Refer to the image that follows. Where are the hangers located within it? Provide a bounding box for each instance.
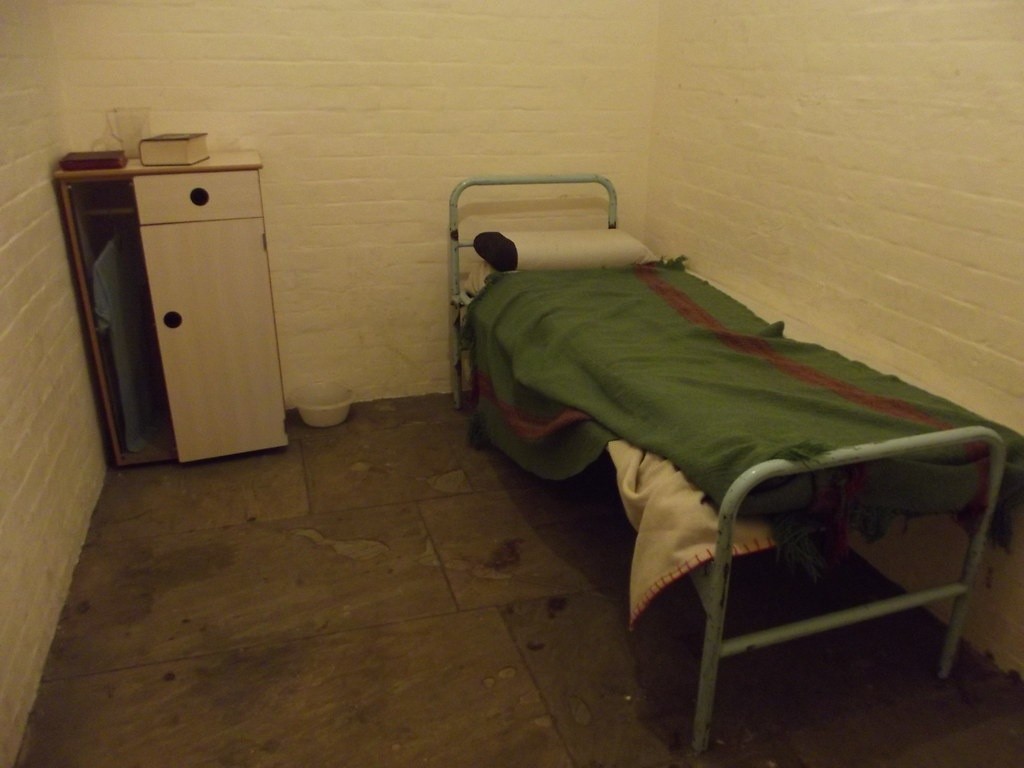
[108,206,124,243]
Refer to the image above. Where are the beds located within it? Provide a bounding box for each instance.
[448,175,1024,755]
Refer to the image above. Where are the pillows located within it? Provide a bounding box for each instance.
[474,229,660,272]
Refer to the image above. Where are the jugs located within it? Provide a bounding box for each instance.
[105,106,154,158]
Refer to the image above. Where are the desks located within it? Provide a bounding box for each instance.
[54,150,289,467]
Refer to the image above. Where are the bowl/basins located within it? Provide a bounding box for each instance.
[289,382,355,427]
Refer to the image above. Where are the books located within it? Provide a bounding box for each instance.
[139,133,210,167]
[59,150,127,170]
